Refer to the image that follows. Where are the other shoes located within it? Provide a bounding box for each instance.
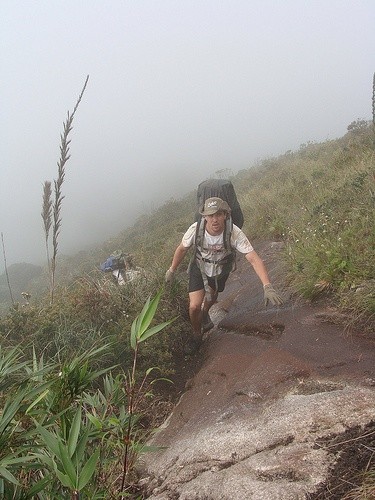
[201,309,214,331]
[184,332,202,354]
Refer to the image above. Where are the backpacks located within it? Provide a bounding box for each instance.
[193,179,244,271]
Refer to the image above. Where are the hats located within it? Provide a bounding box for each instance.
[198,196,232,219]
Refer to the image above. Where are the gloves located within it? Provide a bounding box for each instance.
[262,284,283,306]
[165,269,175,285]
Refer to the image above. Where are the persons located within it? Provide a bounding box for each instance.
[164,196,286,343]
